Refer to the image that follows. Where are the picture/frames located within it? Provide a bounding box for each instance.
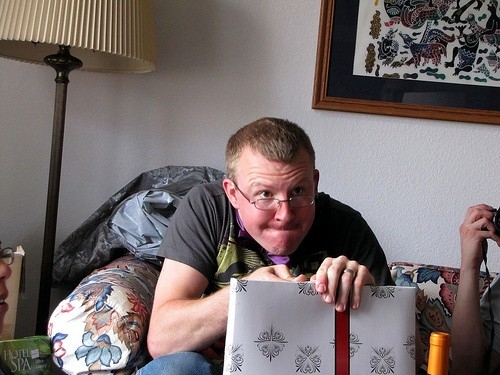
[311,0,500,125]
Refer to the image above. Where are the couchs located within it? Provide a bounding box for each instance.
[46,252,500,375]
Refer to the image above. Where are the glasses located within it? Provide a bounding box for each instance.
[0,247,14,265]
[234,182,316,211]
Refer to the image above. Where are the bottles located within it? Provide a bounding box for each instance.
[427,332,451,375]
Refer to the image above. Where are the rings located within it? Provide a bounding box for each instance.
[344,269,355,278]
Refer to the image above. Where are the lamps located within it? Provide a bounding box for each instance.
[0,0,159,335]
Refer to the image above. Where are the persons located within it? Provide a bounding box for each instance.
[136,118,397,375]
[450,203,500,375]
[0,241,13,375]
